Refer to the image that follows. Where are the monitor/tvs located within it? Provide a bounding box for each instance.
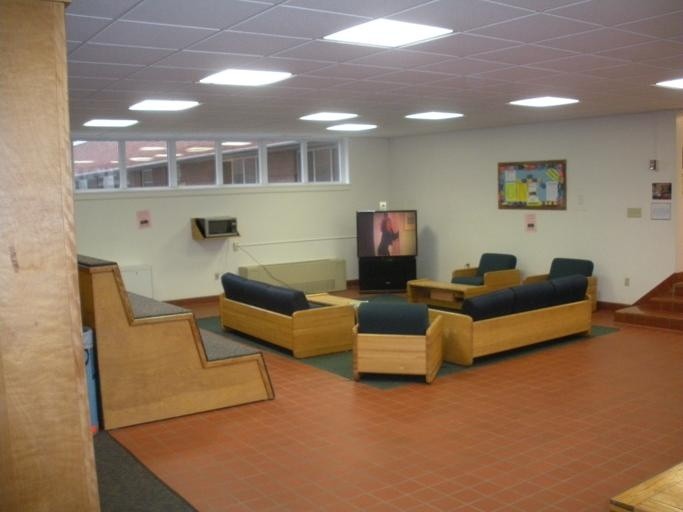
[355,210,418,259]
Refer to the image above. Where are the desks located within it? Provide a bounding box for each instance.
[306,292,358,309]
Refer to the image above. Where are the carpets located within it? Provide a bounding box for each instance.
[94,429,197,512]
[196,294,621,390]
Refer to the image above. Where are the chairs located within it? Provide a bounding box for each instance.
[451,253,521,284]
[522,258,597,312]
[350,301,445,383]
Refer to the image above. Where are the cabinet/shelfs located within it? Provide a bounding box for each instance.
[358,257,417,294]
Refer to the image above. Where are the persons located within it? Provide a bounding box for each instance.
[376,216,398,255]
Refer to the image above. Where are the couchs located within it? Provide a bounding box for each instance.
[219,272,354,360]
[427,275,592,366]
